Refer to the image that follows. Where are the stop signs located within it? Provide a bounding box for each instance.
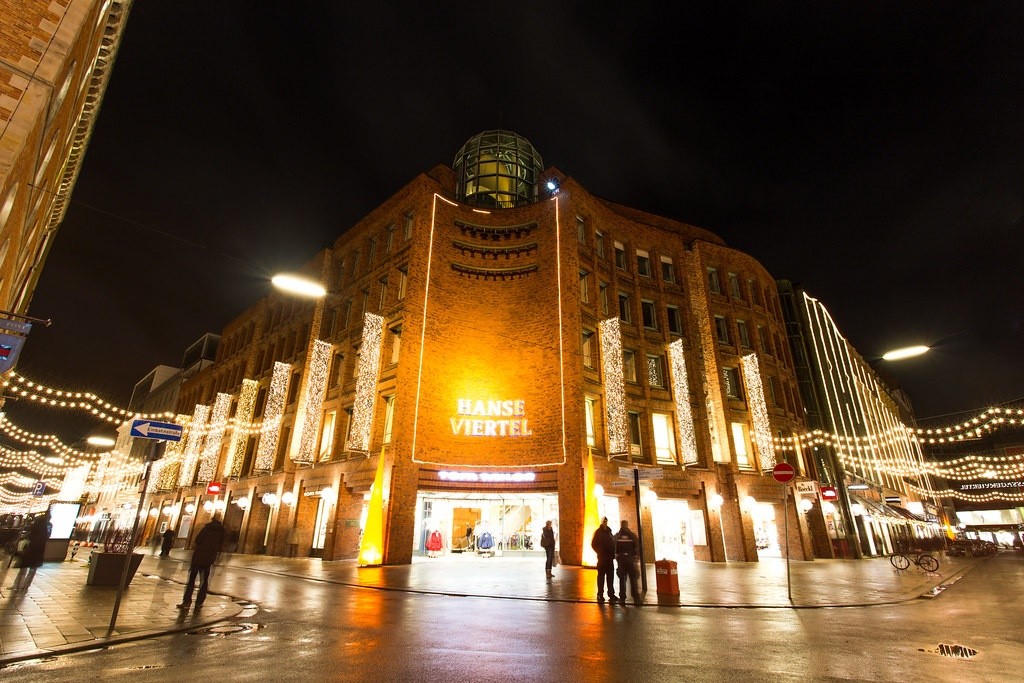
[772,462,796,484]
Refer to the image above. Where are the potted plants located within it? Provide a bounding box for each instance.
[87,528,145,586]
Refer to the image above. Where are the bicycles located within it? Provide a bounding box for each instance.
[890,541,939,573]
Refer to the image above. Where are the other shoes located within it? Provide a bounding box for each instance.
[608,595,618,601]
[597,594,606,601]
[631,593,640,599]
[176,602,205,609]
[620,598,626,603]
[546,569,555,578]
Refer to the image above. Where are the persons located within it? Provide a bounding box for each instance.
[542,521,556,578]
[466,523,472,549]
[591,516,618,602]
[613,520,643,607]
[177,511,226,612]
[6,512,52,590]
[160,526,175,557]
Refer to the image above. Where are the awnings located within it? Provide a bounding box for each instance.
[851,494,923,524]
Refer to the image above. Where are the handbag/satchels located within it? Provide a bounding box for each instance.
[541,538,549,547]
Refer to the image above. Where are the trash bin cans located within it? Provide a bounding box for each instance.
[655,560,680,595]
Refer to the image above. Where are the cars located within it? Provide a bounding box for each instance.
[948,539,997,557]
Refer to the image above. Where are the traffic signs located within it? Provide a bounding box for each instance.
[129,418,184,442]
